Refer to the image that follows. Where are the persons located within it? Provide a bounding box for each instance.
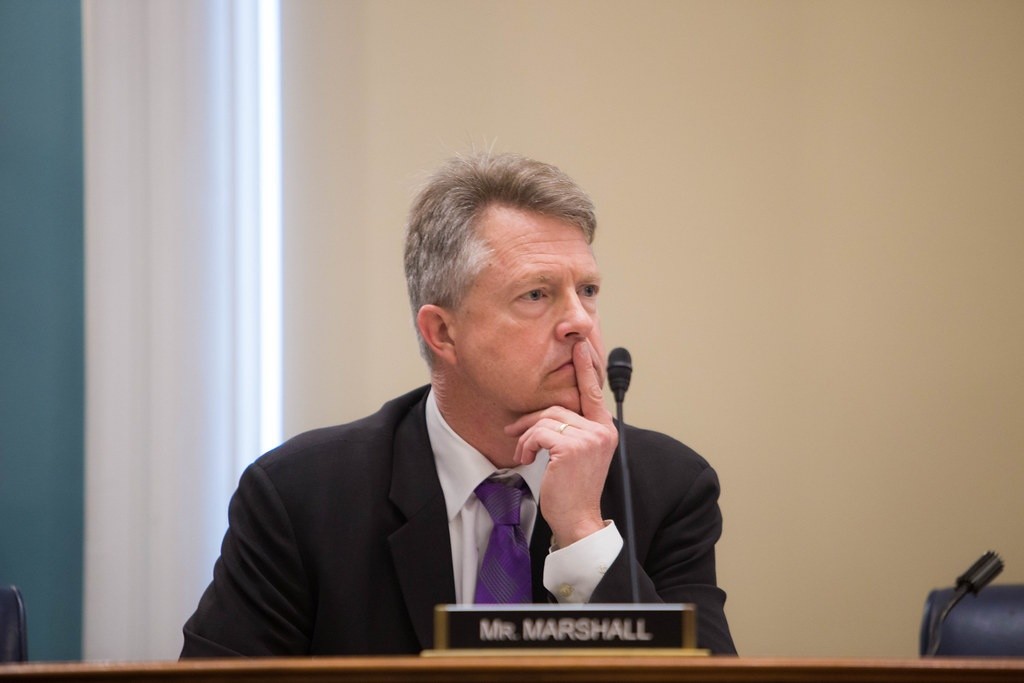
[178,147,740,662]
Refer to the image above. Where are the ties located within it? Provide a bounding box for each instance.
[471,481,534,605]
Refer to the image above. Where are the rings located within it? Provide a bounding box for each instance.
[557,423,568,433]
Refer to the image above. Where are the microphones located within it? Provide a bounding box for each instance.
[929,549,1004,657]
[604,346,639,603]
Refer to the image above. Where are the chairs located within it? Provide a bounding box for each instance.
[920,582,1023,660]
